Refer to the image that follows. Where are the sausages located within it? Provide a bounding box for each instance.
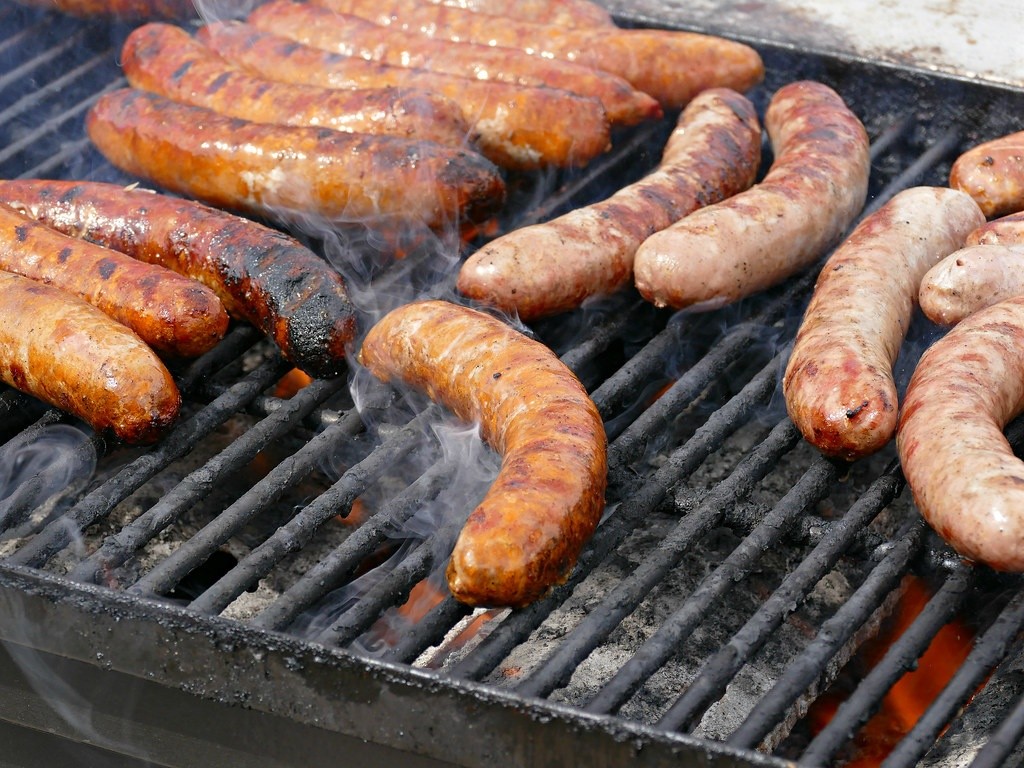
[0,0,1024,605]
